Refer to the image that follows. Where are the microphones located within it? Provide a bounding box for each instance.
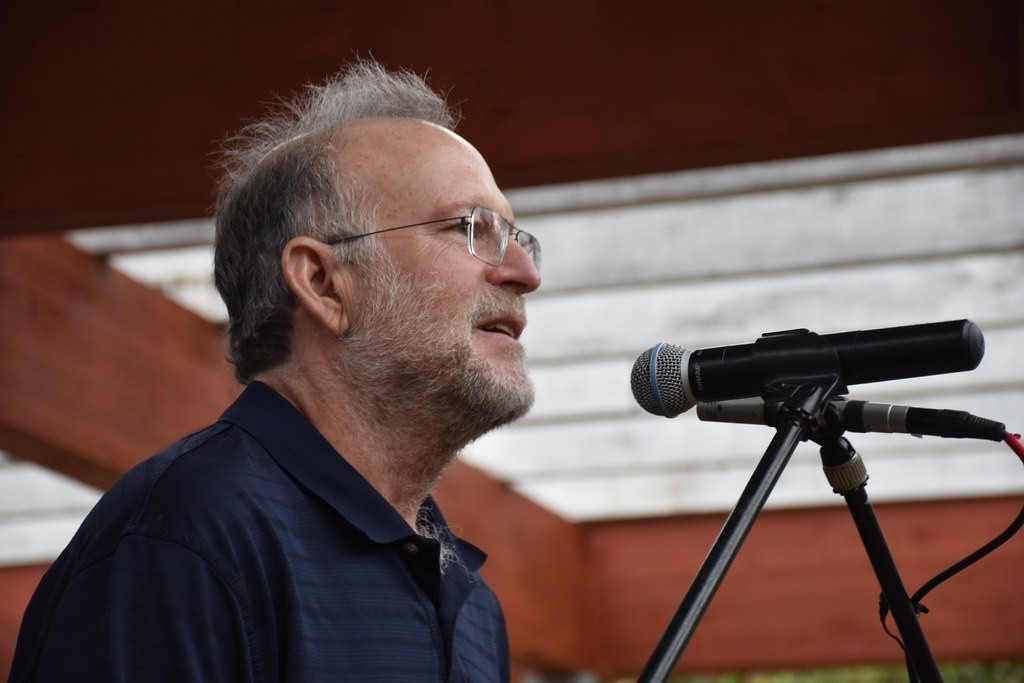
[697,395,1006,441]
[631,318,986,418]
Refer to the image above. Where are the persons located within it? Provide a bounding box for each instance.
[9,49,546,683]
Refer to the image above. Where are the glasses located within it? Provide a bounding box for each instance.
[325,206,542,273]
